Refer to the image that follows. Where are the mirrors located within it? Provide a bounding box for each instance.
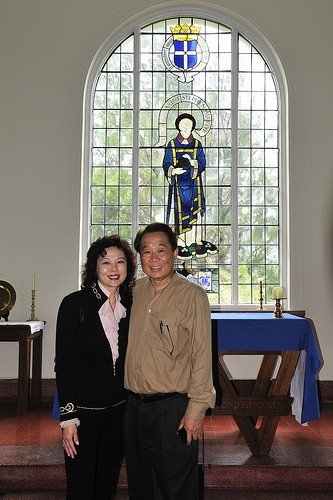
[0,280,16,321]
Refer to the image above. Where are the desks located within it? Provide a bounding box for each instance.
[0,321,46,415]
[212,312,321,456]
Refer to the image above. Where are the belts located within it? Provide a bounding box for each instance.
[129,390,180,403]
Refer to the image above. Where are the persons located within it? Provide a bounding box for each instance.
[54,235,137,500]
[124,223,216,500]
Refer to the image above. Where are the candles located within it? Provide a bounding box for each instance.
[271,286,285,297]
[32,274,35,289]
